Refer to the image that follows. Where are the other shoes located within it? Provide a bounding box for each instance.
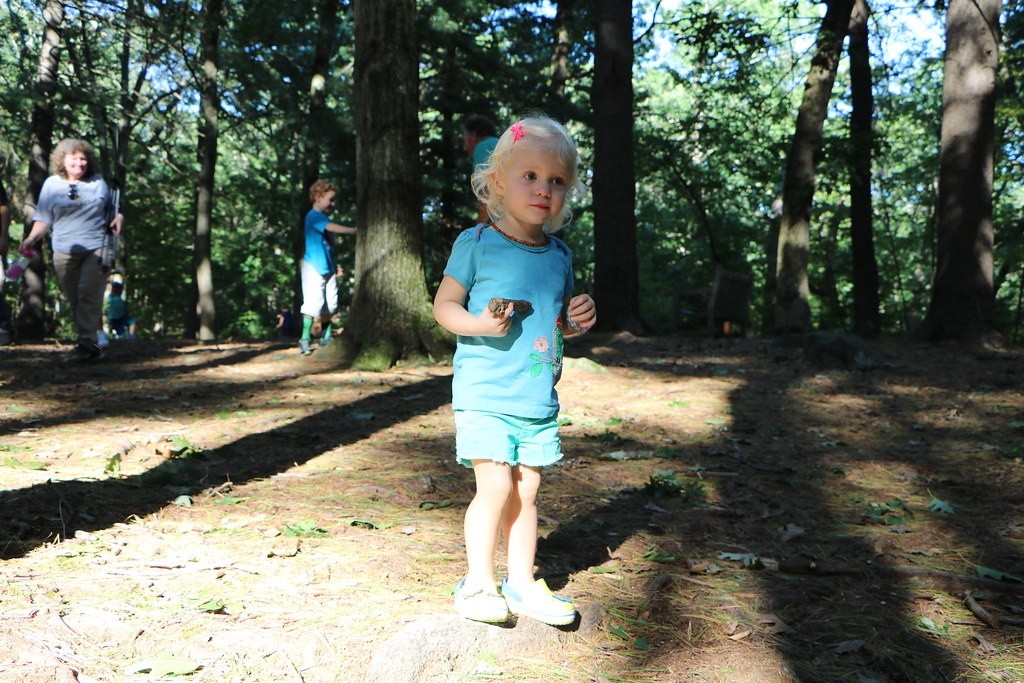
[299,338,312,354]
[319,337,335,345]
[57,344,106,363]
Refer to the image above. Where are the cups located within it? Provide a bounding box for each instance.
[5,249,35,279]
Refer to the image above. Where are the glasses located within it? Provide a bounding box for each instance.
[68,184,78,199]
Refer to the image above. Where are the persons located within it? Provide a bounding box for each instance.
[94,315,110,348]
[0,182,11,300]
[102,268,136,342]
[297,179,358,356]
[17,138,125,363]
[433,116,597,625]
[275,304,295,337]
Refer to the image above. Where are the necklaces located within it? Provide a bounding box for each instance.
[490,221,548,247]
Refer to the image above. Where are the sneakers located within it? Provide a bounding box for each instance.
[507,580,579,625]
[456,576,508,622]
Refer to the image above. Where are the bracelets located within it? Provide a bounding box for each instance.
[27,237,36,241]
[566,306,591,333]
